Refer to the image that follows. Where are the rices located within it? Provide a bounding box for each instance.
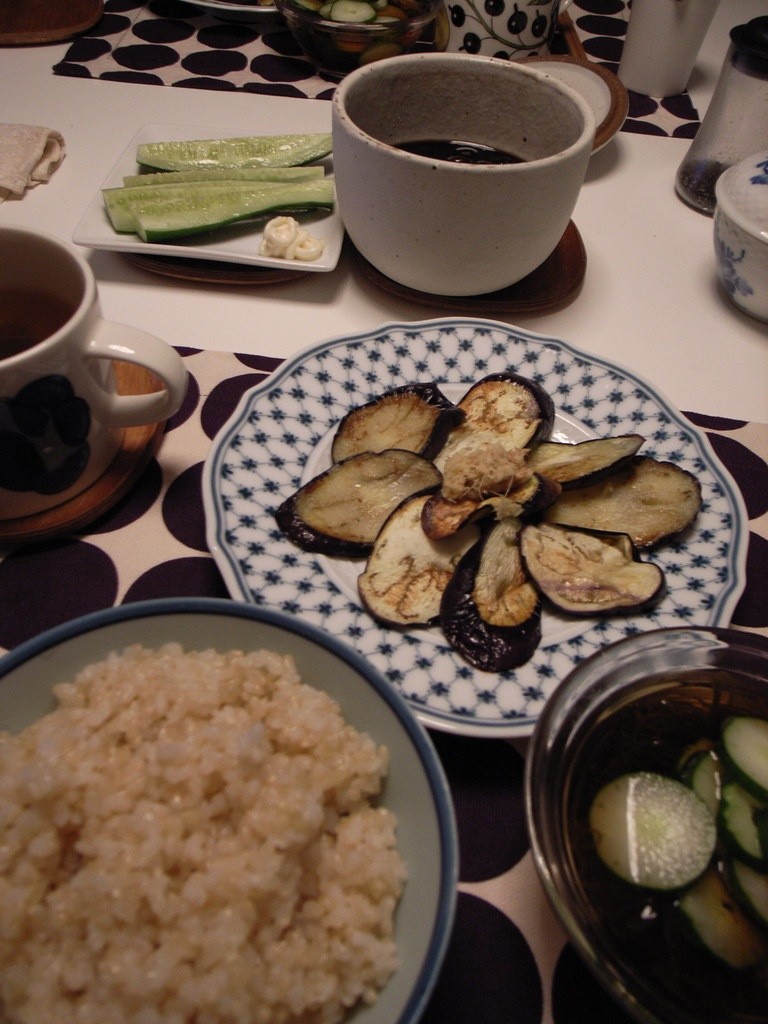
[0,640,408,1024]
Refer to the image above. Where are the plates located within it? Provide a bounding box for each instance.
[120,251,307,284]
[202,318,749,739]
[512,56,629,155]
[73,122,345,272]
[180,0,282,22]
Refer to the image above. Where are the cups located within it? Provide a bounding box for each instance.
[435,0,560,62]
[0,223,188,520]
[616,0,720,97]
[332,53,595,296]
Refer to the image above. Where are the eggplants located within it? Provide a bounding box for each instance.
[275,373,703,675]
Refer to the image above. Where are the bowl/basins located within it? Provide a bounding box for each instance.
[274,0,443,78]
[714,149,768,324]
[0,598,460,1024]
[527,627,768,1024]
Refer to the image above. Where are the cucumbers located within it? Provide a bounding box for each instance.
[588,711,768,981]
[101,133,338,240]
[288,0,400,25]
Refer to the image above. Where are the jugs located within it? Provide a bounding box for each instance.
[676,16,768,212]
